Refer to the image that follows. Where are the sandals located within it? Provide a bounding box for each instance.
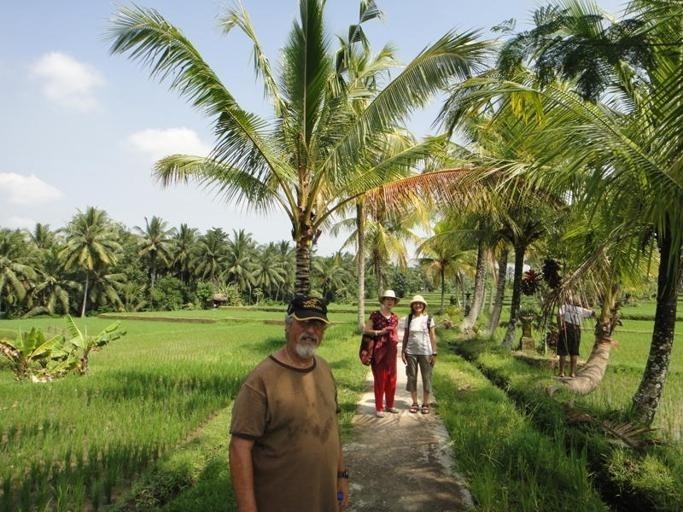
[409,403,430,414]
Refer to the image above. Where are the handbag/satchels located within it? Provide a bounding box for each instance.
[358,333,375,366]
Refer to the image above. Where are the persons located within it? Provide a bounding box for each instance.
[401,295,438,414]
[227,296,350,512]
[556,295,595,378]
[363,289,400,418]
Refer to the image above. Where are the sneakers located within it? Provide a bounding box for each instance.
[375,407,399,417]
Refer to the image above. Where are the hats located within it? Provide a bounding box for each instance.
[286,295,330,326]
[409,295,427,307]
[378,289,400,306]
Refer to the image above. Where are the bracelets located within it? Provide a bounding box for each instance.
[431,353,437,356]
[338,470,349,479]
